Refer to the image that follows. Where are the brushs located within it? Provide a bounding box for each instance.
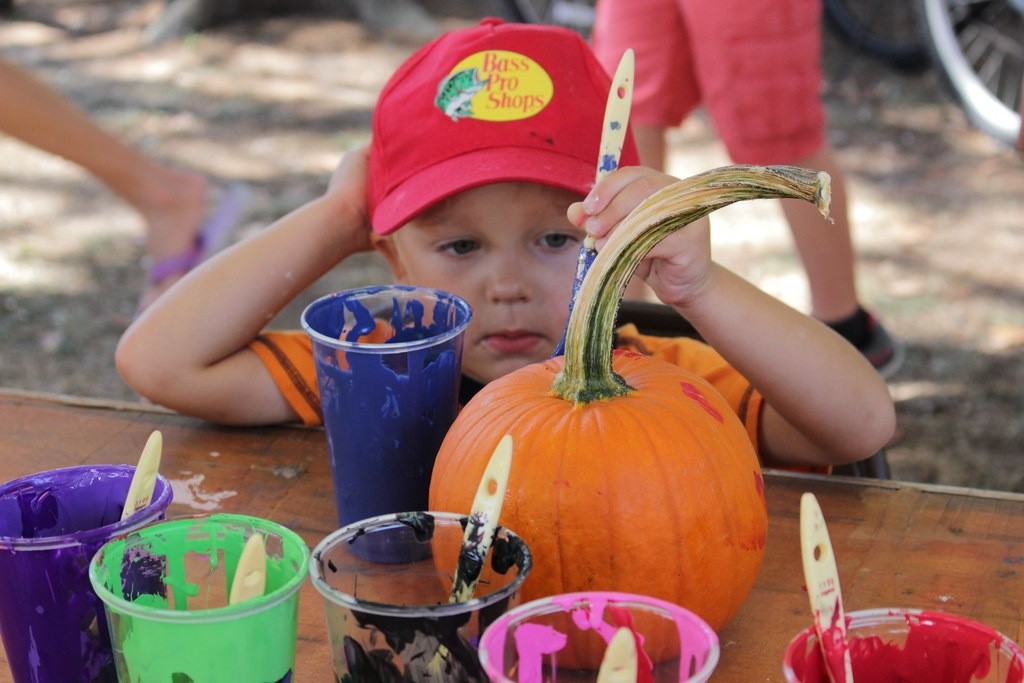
[226,530,268,605]
[798,491,855,683]
[553,45,639,355]
[423,432,514,683]
[595,626,636,683]
[88,426,165,652]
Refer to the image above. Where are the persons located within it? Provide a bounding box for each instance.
[113,15,898,477]
[592,0,906,381]
[0,57,254,323]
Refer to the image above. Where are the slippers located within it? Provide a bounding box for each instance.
[135,182,252,320]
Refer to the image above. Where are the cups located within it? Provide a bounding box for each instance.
[0,463,719,683]
[300,283,472,563]
[782,606,1024,683]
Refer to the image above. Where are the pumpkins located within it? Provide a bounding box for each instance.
[429,160,835,668]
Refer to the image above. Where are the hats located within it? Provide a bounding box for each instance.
[367,17,645,248]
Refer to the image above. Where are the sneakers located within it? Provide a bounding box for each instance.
[825,304,907,382]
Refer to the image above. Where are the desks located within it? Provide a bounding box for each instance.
[0,385,1024,682]
